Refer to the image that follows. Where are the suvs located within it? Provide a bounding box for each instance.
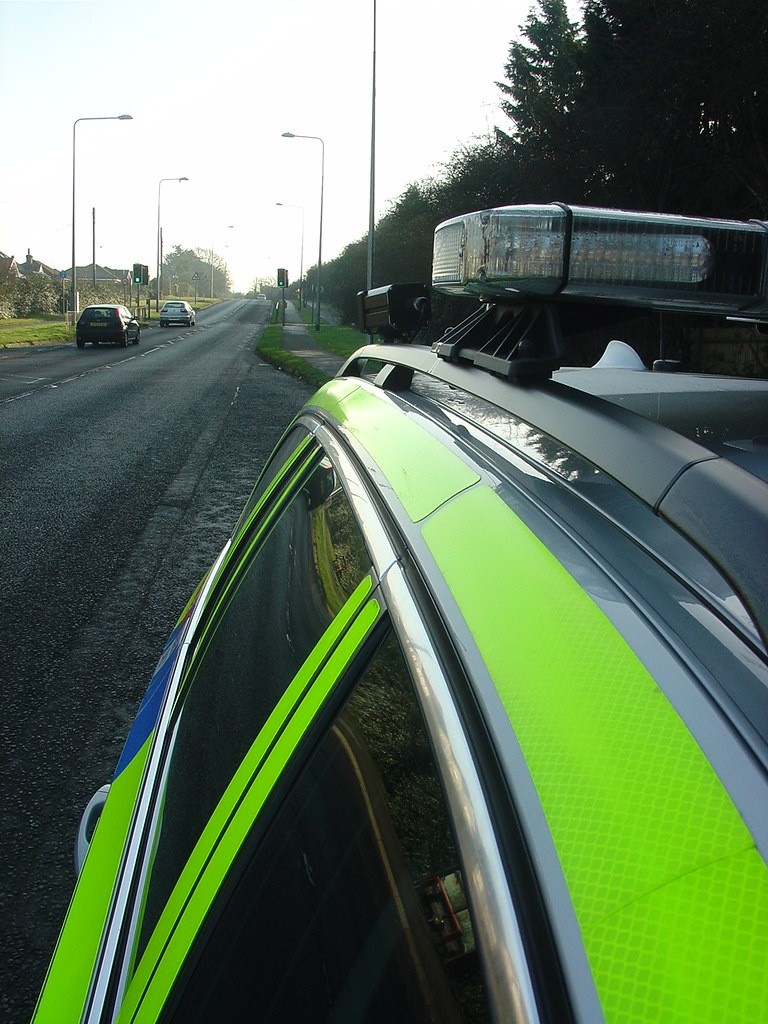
[76,305,141,349]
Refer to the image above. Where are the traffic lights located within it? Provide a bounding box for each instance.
[277,267,287,288]
[132,263,144,284]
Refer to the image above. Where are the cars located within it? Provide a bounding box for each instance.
[157,300,197,327]
[257,294,266,300]
[29,203,768,1023]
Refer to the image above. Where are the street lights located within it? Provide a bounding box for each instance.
[153,178,188,312]
[280,133,321,330]
[66,113,134,311]
[275,204,304,313]
[210,225,235,299]
